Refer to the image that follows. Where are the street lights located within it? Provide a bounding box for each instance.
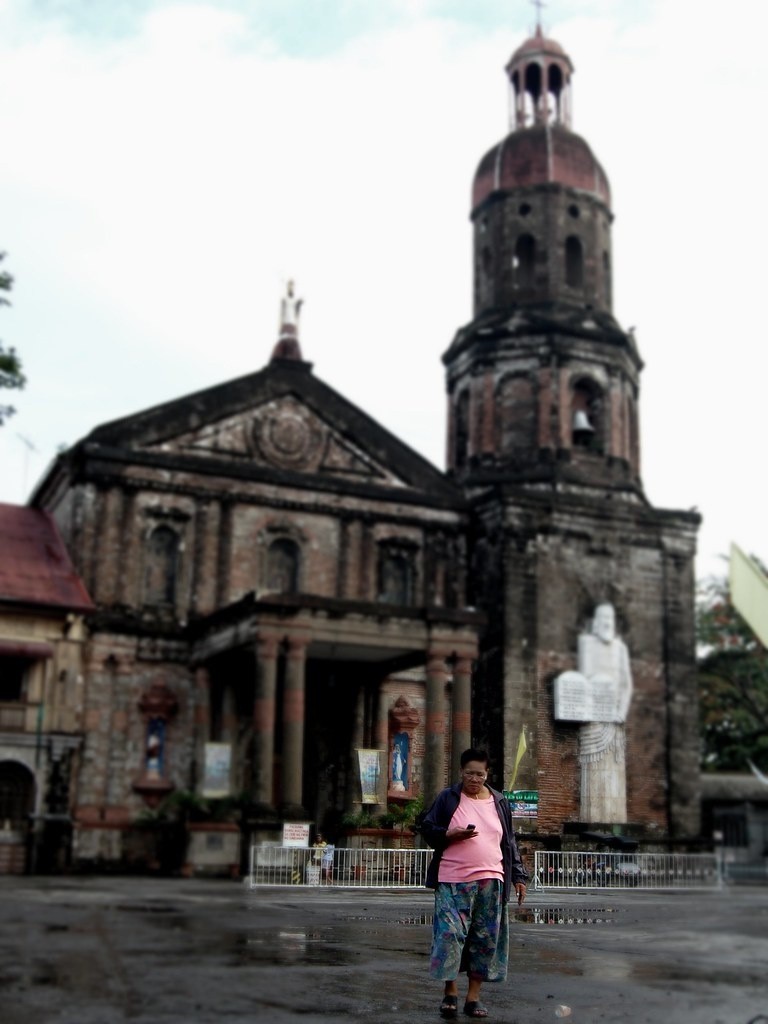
[29,614,89,828]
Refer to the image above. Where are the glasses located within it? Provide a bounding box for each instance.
[462,770,487,781]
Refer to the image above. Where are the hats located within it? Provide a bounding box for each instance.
[316,834,322,837]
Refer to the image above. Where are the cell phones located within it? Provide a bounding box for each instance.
[466,824,476,830]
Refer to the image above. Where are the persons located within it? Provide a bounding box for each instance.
[313,834,335,885]
[420,750,528,1017]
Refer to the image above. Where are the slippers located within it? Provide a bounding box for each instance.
[463,1000,488,1018]
[441,995,457,1014]
